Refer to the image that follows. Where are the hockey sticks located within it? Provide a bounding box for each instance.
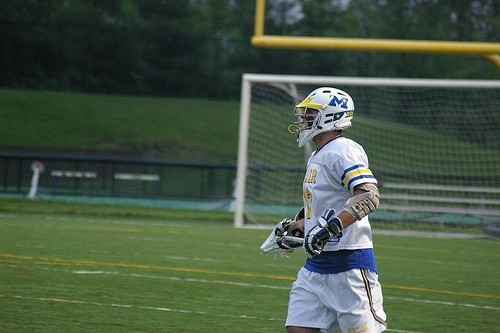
[260,218,340,252]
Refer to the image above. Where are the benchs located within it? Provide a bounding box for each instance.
[114,173,159,197]
[50,170,97,195]
[377,182,500,229]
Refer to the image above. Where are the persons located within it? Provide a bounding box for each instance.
[275,87,387,333]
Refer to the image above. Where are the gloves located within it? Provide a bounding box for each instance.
[303,208,343,259]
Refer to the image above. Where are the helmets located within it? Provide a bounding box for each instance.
[294,87,355,147]
